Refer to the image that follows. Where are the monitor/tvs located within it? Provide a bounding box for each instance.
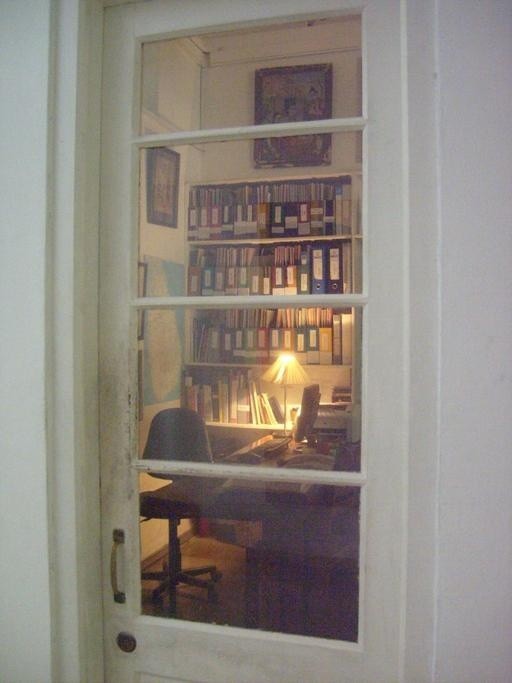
[292,382,321,453]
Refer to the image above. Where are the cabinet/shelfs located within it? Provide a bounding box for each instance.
[180,173,360,432]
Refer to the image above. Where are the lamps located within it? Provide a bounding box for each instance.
[260,351,307,438]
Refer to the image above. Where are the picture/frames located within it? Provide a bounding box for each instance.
[253,62,333,171]
[145,146,181,229]
[138,262,147,421]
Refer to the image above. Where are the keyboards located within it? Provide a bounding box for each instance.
[253,437,292,457]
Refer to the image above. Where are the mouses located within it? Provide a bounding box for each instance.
[242,451,262,463]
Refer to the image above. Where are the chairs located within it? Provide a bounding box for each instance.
[138,408,360,642]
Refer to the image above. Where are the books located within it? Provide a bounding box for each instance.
[292,388,352,425]
[188,245,343,296]
[191,307,352,365]
[184,366,285,425]
[189,177,351,241]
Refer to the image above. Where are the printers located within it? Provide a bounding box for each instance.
[290,403,361,445]
